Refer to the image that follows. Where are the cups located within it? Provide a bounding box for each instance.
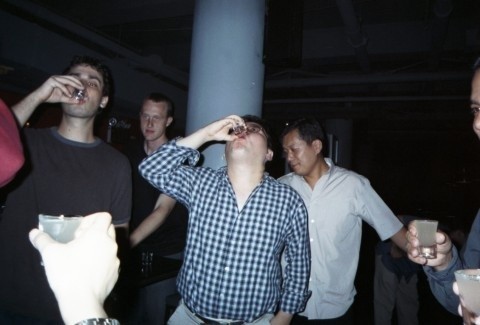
[231,123,246,138]
[72,71,90,101]
[454,268,480,325]
[37,214,85,266]
[413,219,439,262]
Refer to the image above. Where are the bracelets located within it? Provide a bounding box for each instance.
[74,318,119,325]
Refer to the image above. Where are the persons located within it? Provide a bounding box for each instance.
[275,115,408,325]
[138,114,312,325]
[117,92,188,325]
[28,211,120,325]
[406,56,480,325]
[373,215,429,325]
[0,54,132,325]
